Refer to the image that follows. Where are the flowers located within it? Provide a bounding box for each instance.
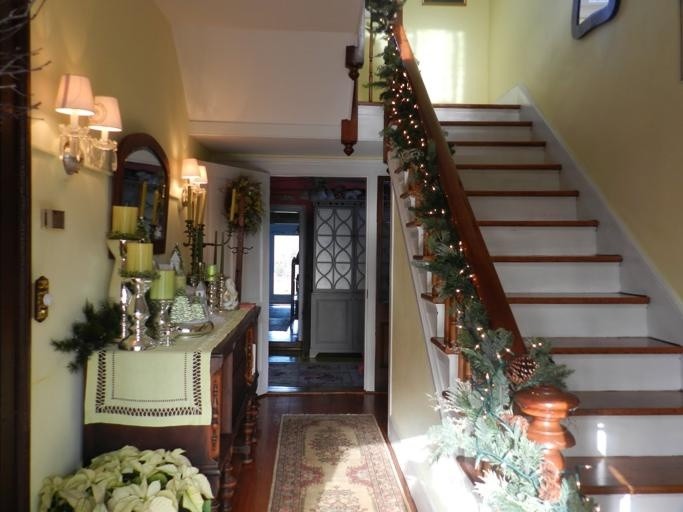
[38,443,214,512]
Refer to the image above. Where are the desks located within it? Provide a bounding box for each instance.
[84,302,261,512]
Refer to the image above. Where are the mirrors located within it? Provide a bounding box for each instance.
[110,132,169,258]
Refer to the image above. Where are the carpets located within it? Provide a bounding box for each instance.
[266,413,417,512]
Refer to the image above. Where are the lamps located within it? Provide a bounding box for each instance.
[181,158,200,205]
[53,74,94,176]
[89,96,123,171]
[192,166,209,194]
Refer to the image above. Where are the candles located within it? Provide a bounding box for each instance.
[188,186,192,220]
[195,194,201,226]
[127,242,153,272]
[139,180,147,217]
[113,206,138,236]
[199,191,206,224]
[151,269,174,300]
[176,275,187,289]
[192,192,194,221]
[151,191,159,225]
[204,265,218,281]
[230,188,236,221]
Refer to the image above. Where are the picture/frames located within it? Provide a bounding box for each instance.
[571,0,620,41]
